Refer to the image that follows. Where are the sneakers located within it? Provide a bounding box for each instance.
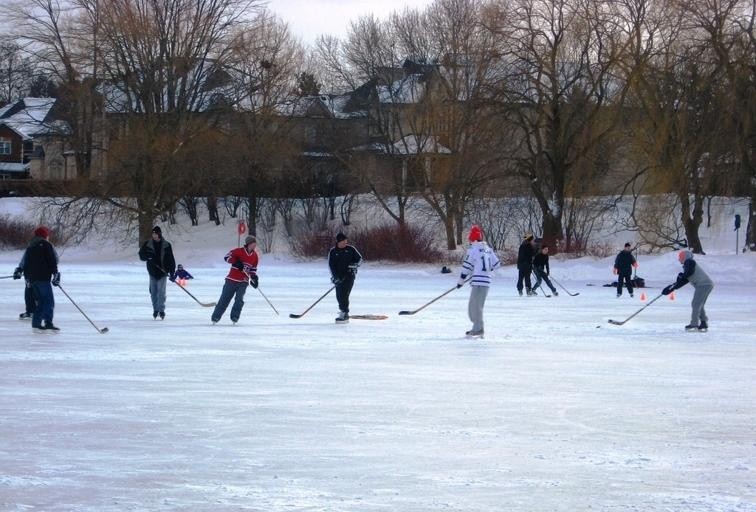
[519,288,537,295]
[686,323,707,329]
[33,324,59,330]
[335,313,349,321]
[617,294,634,297]
[21,313,33,318]
[466,331,483,336]
[153,311,165,317]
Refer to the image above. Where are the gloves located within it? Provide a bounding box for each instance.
[234,261,243,271]
[52,273,60,286]
[250,275,258,288]
[14,268,22,279]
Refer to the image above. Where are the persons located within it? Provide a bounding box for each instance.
[603,275,645,288]
[517,232,538,295]
[22,227,59,331]
[211,236,259,322]
[613,243,640,298]
[532,245,558,295]
[138,225,175,317]
[175,263,193,280]
[662,249,715,329]
[457,226,501,335]
[13,254,37,319]
[328,233,363,321]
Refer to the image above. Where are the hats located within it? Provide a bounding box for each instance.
[523,233,532,239]
[153,226,161,234]
[246,236,255,244]
[469,226,481,240]
[35,227,48,238]
[336,234,346,241]
[683,251,692,260]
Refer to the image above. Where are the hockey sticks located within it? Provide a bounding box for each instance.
[56,283,112,335]
[607,289,664,326]
[154,260,217,309]
[530,274,552,300]
[395,277,476,317]
[548,274,582,299]
[290,275,347,322]
